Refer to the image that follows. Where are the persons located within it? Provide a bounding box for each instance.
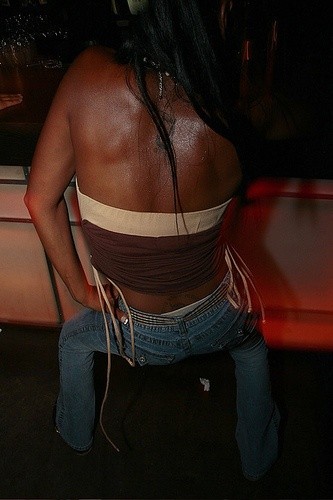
[24,0,288,484]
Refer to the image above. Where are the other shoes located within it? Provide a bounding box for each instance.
[55,417,93,456]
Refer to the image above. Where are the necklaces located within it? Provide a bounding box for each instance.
[143,57,184,101]
